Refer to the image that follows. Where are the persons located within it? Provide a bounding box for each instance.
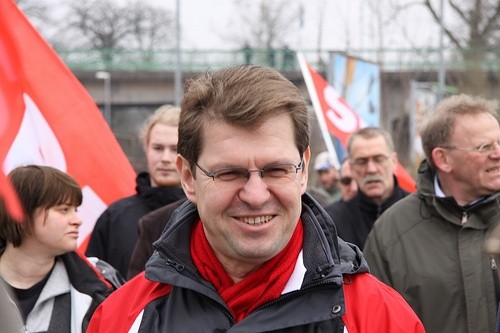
[84,104,415,283]
[361,95,500,333]
[87,62,427,333]
[0,165,126,333]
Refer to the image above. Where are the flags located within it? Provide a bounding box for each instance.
[306,67,418,194]
[0,0,141,286]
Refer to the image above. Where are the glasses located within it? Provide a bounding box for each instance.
[335,176,353,185]
[441,140,500,153]
[193,158,305,189]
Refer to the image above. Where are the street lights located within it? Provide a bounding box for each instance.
[95,71,111,125]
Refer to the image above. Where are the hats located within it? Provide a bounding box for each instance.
[313,151,341,171]
